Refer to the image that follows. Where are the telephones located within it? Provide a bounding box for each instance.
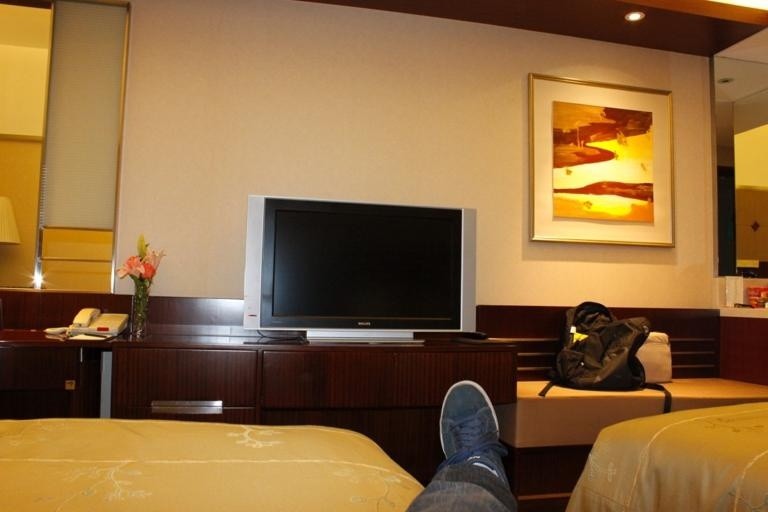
[68,308,129,336]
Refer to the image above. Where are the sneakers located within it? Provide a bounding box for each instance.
[437,379,509,485]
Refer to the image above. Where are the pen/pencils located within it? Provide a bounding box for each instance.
[85,333,106,338]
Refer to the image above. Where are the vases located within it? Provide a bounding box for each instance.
[126,296,149,342]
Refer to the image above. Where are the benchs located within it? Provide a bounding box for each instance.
[474,303,767,449]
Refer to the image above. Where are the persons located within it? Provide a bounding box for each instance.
[407,377,521,511]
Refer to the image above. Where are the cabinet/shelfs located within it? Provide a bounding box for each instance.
[1,325,514,489]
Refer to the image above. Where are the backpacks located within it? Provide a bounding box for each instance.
[551,300,650,394]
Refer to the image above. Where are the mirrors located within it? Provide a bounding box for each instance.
[713,28,768,279]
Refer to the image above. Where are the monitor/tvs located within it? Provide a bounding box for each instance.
[242,194,475,341]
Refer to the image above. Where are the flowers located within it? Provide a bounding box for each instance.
[117,234,166,322]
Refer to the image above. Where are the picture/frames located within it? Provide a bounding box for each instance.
[525,72,677,249]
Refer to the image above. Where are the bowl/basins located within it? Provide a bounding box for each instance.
[747,287,767,308]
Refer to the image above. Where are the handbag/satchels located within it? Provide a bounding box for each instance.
[638,331,672,384]
